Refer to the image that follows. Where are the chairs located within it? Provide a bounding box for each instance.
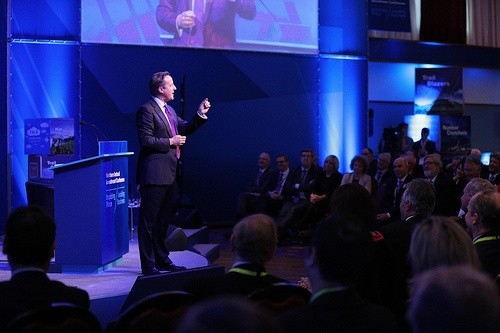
[108,290,201,333]
[2,302,105,333]
[247,282,313,318]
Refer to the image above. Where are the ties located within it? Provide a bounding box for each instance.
[299,170,306,185]
[397,179,401,192]
[164,104,180,159]
[377,172,381,181]
[276,173,283,192]
[189,0,204,46]
[490,175,494,182]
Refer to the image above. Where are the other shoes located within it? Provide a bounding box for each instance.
[159,264,186,271]
[142,267,161,275]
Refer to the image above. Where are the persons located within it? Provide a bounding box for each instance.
[412,127,436,154]
[156,0,257,50]
[170,148,500,333]
[0,206,90,328]
[393,122,413,152]
[134,70,212,277]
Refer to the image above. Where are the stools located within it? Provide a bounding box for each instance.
[128,198,142,243]
